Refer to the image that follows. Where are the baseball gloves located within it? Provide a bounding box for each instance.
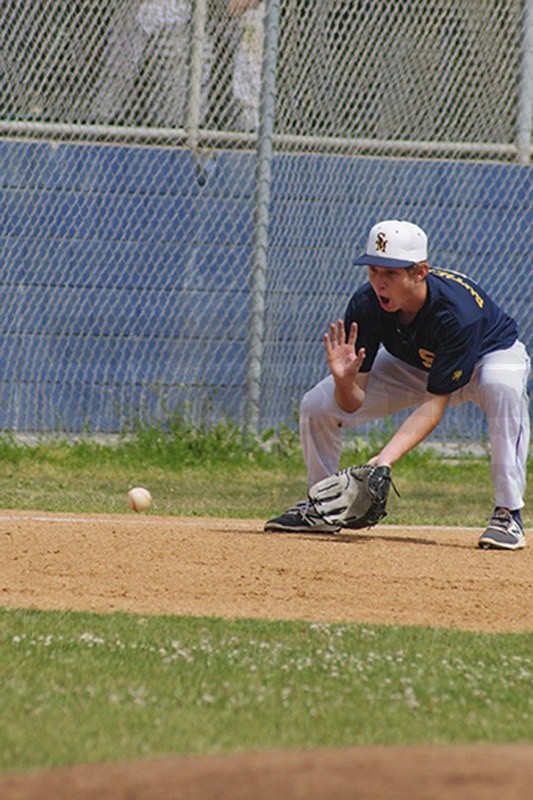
[308,462,392,530]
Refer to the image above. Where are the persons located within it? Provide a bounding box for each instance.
[89,0,262,131]
[263,219,531,551]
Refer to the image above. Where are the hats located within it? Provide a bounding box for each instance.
[354,219,429,268]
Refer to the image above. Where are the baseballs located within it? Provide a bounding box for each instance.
[127,487,151,513]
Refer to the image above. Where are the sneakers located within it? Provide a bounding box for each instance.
[264,500,346,533]
[479,510,527,550]
